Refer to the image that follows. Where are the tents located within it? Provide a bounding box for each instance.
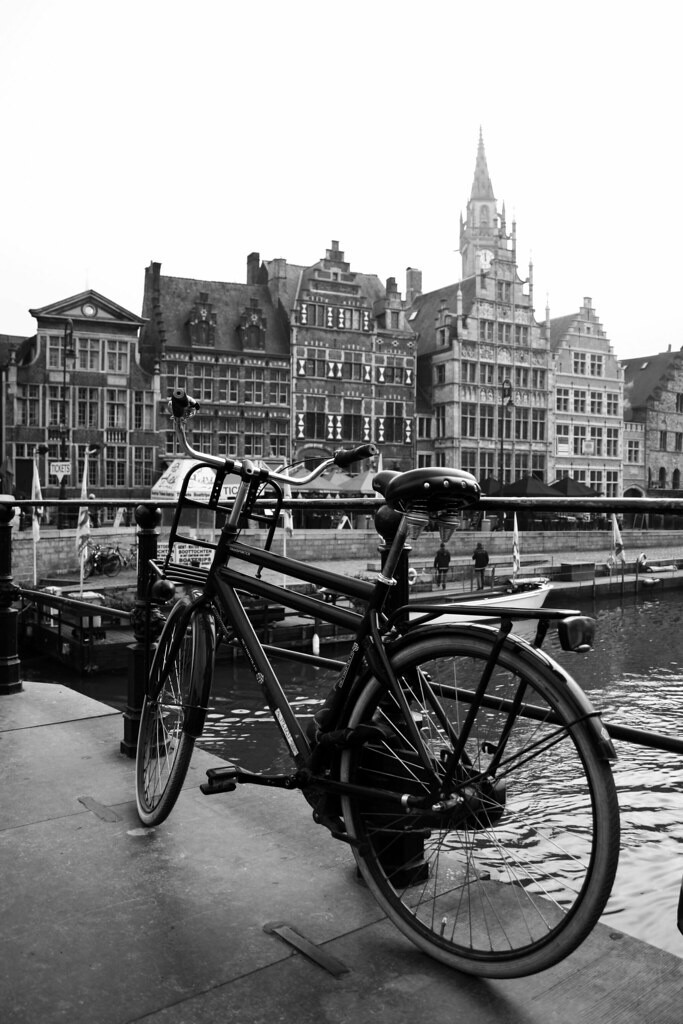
[150,458,377,500]
[478,476,598,497]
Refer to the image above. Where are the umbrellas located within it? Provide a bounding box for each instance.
[0,456,14,494]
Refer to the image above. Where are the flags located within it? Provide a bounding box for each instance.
[513,516,520,573]
[32,467,43,541]
[76,482,91,563]
[613,516,625,561]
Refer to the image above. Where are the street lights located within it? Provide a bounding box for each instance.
[60,318,79,500]
[500,379,514,497]
[135,386,621,982]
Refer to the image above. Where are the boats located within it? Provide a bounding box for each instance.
[409,582,555,625]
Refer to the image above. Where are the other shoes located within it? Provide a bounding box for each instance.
[437,583,440,586]
[477,587,483,590]
[443,583,445,589]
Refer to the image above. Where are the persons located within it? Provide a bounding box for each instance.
[88,493,101,528]
[617,516,623,531]
[434,543,450,589]
[471,543,489,590]
[598,516,605,531]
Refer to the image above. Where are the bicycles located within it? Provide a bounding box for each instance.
[83,541,139,580]
